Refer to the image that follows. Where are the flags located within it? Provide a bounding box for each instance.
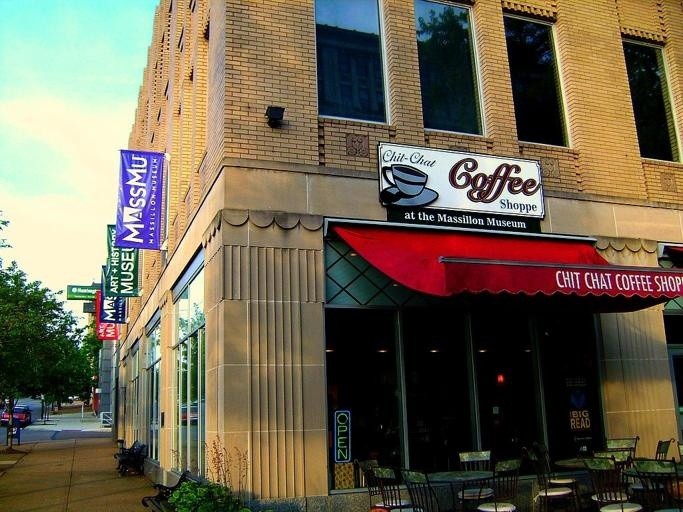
[113,150,168,251]
[104,223,141,299]
[99,264,130,326]
[93,290,119,341]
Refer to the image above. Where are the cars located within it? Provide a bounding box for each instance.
[177,399,204,426]
[0,396,33,426]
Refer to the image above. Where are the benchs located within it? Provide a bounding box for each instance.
[142,471,224,512]
[113,440,147,477]
